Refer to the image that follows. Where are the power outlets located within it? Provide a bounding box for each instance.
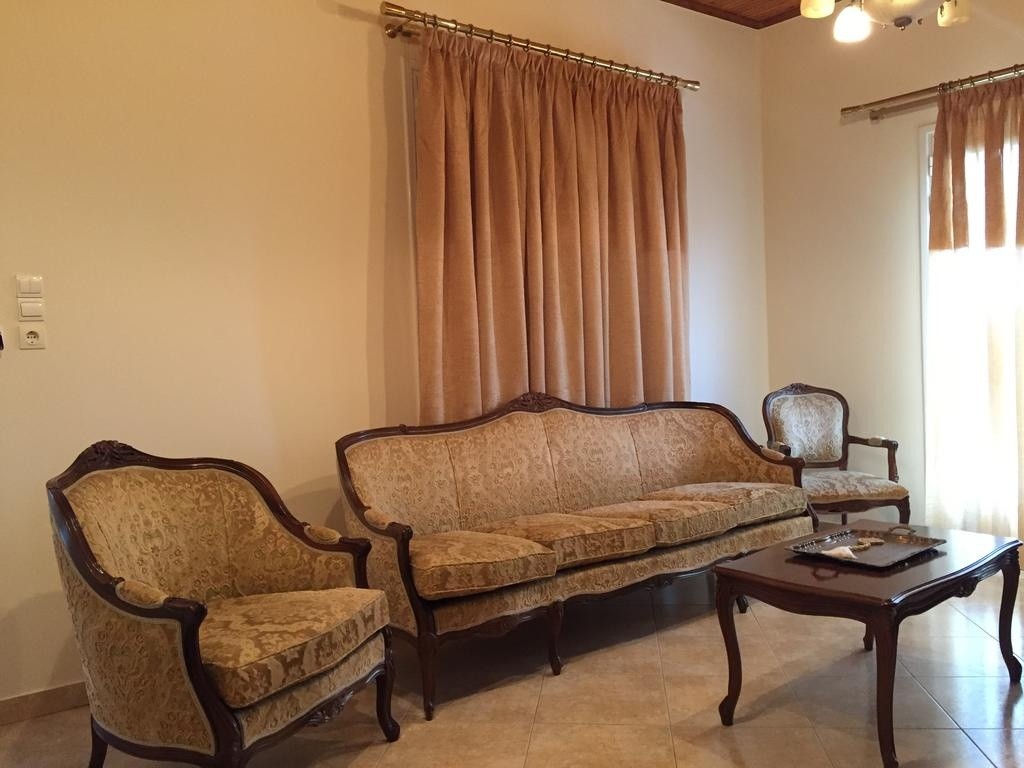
[19,324,48,350]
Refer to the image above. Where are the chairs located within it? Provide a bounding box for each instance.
[762,383,911,527]
[46,439,400,768]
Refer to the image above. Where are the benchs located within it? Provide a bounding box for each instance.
[336,392,818,722]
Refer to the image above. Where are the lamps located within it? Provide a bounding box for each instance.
[799,0,973,43]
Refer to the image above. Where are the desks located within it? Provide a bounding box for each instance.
[711,518,1023,768]
